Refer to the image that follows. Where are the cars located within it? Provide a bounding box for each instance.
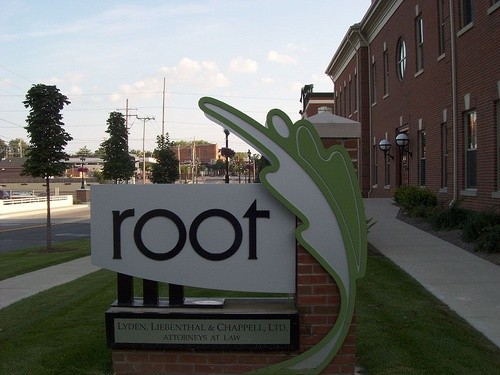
[5,190,37,205]
[0,189,10,200]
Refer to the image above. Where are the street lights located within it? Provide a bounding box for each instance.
[247,150,252,183]
[223,128,230,183]
[80,156,86,189]
